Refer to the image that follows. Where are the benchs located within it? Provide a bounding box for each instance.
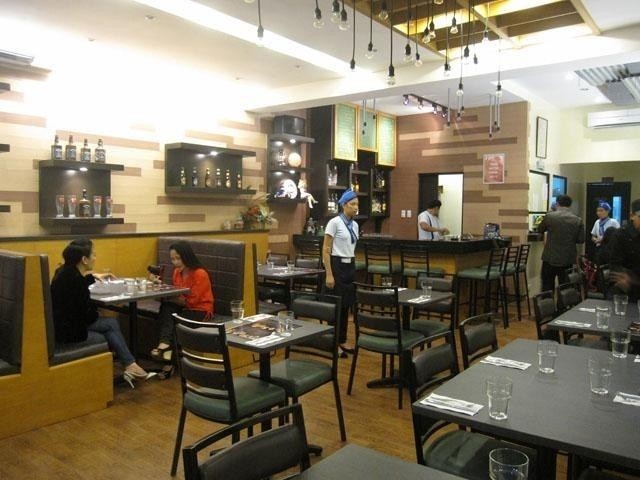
[189,235,288,317]
[3,249,113,407]
[0,251,28,407]
[118,237,248,372]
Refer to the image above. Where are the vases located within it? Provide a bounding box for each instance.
[242,221,267,231]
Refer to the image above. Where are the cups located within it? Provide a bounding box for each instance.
[93,195,102,218]
[124,278,136,297]
[55,194,65,218]
[596,303,611,330]
[278,310,294,337]
[536,340,559,375]
[230,299,244,324]
[614,294,628,315]
[105,195,113,218]
[486,375,512,421]
[266,257,275,270]
[421,279,433,298]
[67,194,77,218]
[588,353,610,395]
[135,277,147,294]
[609,327,631,359]
[489,448,529,480]
[381,277,393,293]
[287,260,295,273]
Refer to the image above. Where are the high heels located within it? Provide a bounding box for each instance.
[123,369,158,389]
[158,364,176,380]
[150,343,175,360]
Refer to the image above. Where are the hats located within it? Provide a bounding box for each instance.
[336,188,357,207]
[596,200,611,210]
[551,201,557,210]
[631,199,639,217]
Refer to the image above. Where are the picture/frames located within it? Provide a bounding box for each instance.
[535,117,548,158]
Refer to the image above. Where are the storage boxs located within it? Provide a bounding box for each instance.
[273,114,306,136]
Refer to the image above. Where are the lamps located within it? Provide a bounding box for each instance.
[445,89,466,127]
[473,2,480,71]
[402,93,451,119]
[403,0,413,65]
[348,2,358,77]
[386,0,397,86]
[456,0,464,97]
[379,0,388,22]
[338,1,349,31]
[256,0,266,49]
[414,0,422,70]
[495,36,503,98]
[330,2,340,23]
[422,0,431,45]
[462,1,472,66]
[443,0,451,79]
[450,0,458,35]
[482,24,489,50]
[428,0,437,40]
[312,0,325,30]
[488,93,503,140]
[365,0,376,59]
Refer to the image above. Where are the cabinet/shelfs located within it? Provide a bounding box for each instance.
[165,142,257,196]
[267,133,318,205]
[308,102,396,221]
[37,159,125,227]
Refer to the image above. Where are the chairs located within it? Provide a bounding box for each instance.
[406,329,534,480]
[456,247,508,329]
[401,245,446,322]
[269,255,323,324]
[460,313,557,480]
[183,402,312,480]
[390,270,459,389]
[258,252,290,303]
[170,312,287,477]
[556,281,612,352]
[495,243,532,321]
[533,291,561,345]
[475,246,521,329]
[247,292,348,443]
[346,281,432,410]
[353,260,372,324]
[299,239,330,292]
[363,243,410,317]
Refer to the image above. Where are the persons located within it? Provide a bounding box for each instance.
[592,199,640,302]
[537,194,584,315]
[151,240,213,379]
[321,189,360,358]
[590,201,620,266]
[417,198,450,241]
[50,238,157,388]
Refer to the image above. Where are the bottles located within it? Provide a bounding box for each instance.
[354,175,359,191]
[328,191,332,212]
[159,264,166,281]
[177,166,186,188]
[329,165,339,186]
[225,168,232,189]
[95,139,105,163]
[318,225,326,235]
[79,189,90,217]
[236,170,242,191]
[372,193,387,213]
[307,218,315,234]
[330,192,336,211]
[51,134,62,160]
[80,138,91,161]
[205,168,214,187]
[65,135,76,160]
[192,166,198,186]
[334,192,340,212]
[375,170,386,189]
[216,168,222,189]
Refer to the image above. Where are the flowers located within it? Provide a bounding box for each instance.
[238,203,279,224]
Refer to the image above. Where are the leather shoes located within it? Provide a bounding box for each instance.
[340,342,356,354]
[337,345,349,359]
